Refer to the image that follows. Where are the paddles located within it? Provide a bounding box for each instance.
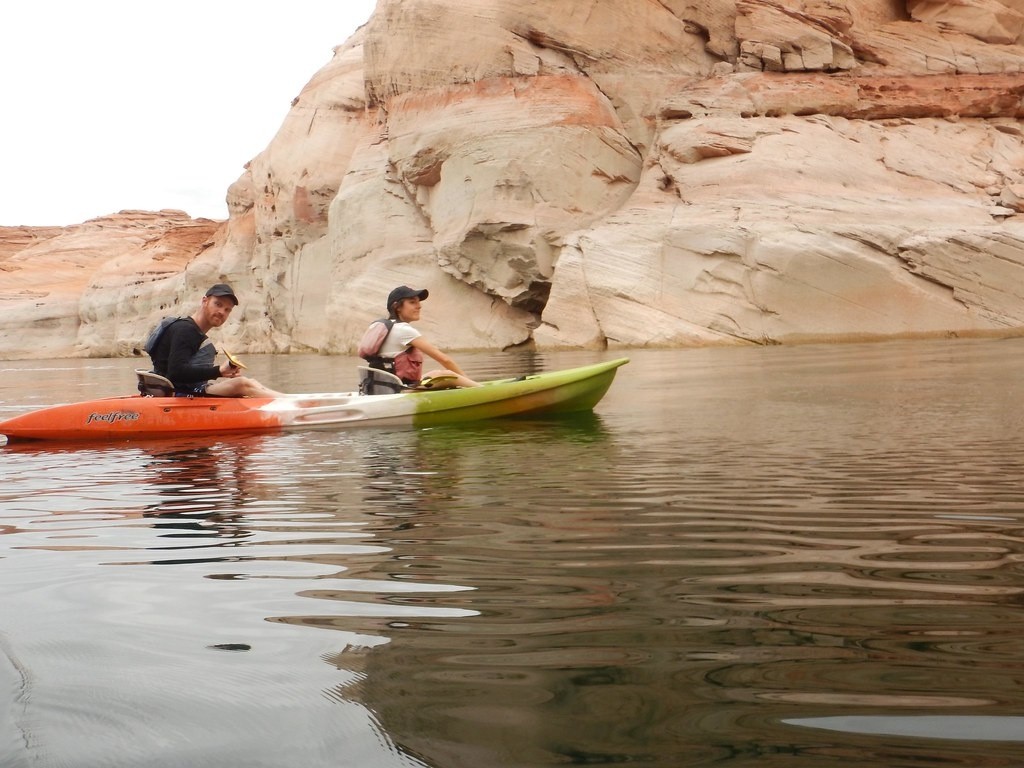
[220,345,248,370]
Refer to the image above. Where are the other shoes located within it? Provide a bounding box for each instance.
[515,375,527,381]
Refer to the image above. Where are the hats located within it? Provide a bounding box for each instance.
[387,285,429,307]
[206,284,238,305]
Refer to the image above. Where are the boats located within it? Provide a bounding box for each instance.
[0,355,632,443]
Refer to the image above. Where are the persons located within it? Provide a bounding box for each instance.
[369,286,483,393]
[152,284,288,399]
